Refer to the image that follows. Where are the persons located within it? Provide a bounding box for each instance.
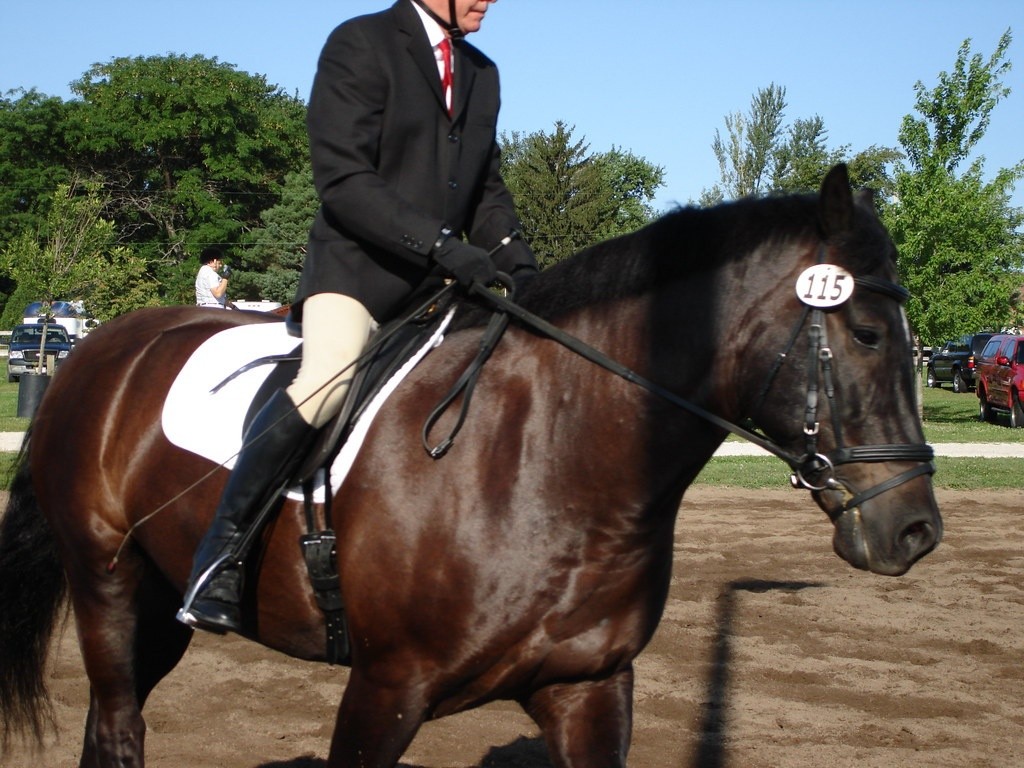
[194,244,241,310]
[181,0,539,635]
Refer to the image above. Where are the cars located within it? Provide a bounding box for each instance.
[0,323,76,383]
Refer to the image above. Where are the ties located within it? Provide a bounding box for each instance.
[437,39,454,119]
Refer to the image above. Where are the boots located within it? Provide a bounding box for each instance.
[186,388,318,629]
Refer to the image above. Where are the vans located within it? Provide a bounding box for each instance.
[912,346,948,368]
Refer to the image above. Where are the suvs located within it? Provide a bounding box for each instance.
[926,332,1006,393]
[974,333,1024,429]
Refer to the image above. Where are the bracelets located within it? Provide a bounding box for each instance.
[232,305,235,309]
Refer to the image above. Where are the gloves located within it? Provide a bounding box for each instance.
[432,236,497,287]
[223,265,232,279]
[232,306,239,311]
[511,267,539,282]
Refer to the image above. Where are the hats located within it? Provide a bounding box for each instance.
[200,247,225,263]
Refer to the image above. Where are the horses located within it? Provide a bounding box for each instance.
[0,163,943,768]
